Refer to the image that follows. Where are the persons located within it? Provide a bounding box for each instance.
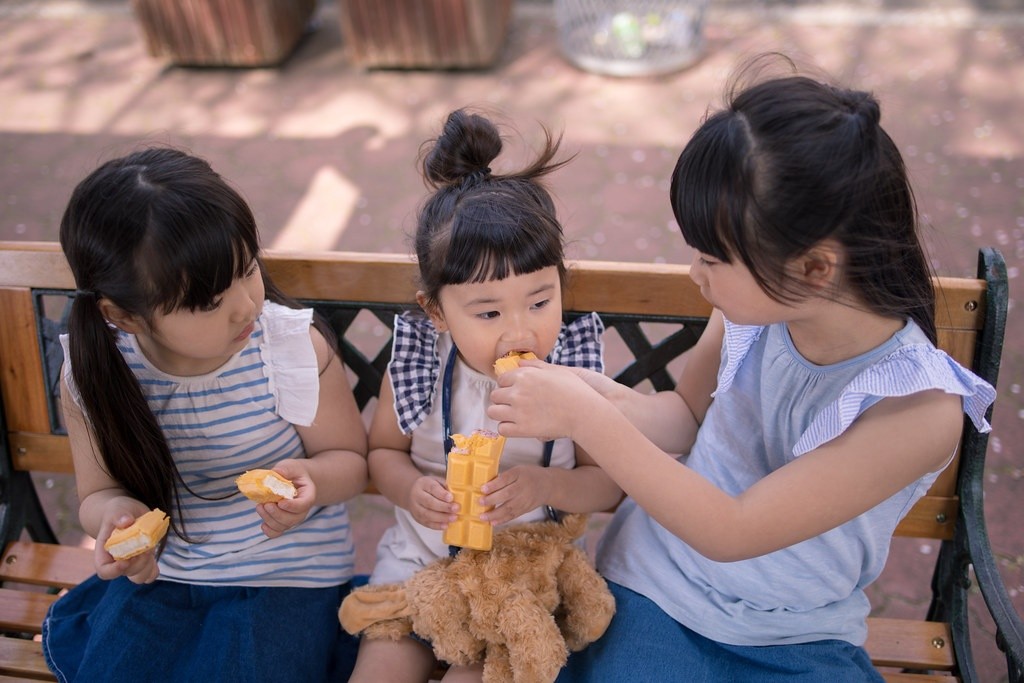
[39,146,372,683]
[483,50,997,683]
[347,106,626,682]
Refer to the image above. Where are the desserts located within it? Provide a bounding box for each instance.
[444,433,504,551]
[105,509,170,563]
[494,351,536,374]
[235,470,297,505]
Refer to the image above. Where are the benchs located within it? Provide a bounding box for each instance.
[0,237,1024,683]
